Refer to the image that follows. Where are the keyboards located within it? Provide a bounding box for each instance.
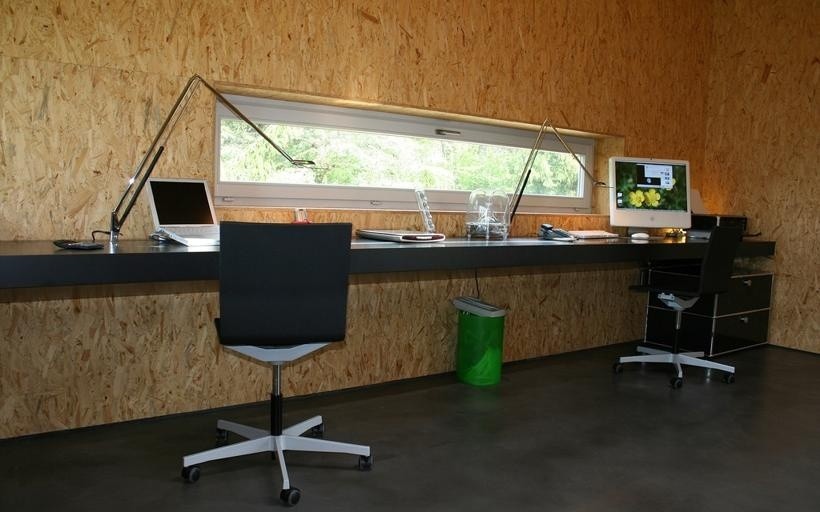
[568,229,619,239]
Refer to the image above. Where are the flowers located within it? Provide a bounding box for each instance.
[628,188,662,209]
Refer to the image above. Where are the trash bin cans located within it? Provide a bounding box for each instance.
[456,311,504,385]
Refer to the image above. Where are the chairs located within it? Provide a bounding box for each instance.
[609,226,745,391]
[180,221,376,505]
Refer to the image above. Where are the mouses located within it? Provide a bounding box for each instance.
[631,233,649,238]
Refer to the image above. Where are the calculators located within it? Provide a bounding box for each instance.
[53,240,104,250]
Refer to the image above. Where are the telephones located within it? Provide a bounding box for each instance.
[540,224,575,242]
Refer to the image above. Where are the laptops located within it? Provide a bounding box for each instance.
[145,177,220,246]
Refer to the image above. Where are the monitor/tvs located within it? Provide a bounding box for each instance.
[609,156,692,239]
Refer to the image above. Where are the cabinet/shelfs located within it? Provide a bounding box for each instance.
[642,263,775,358]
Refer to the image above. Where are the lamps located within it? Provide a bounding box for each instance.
[505,117,615,224]
[105,72,317,242]
